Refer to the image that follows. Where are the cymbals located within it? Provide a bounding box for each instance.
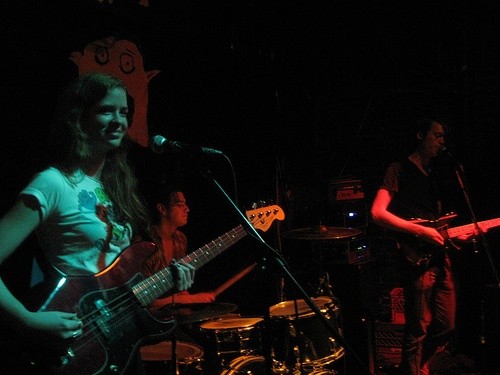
[166,300,239,322]
[278,225,363,242]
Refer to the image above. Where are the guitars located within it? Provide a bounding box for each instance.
[0,199,286,375]
[395,212,500,265]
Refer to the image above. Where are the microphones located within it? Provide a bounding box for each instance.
[151,135,223,155]
[441,146,462,168]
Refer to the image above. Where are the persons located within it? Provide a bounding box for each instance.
[369,118,488,375]
[0,70,196,375]
[149,188,217,310]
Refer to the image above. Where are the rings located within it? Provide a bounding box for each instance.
[71,329,78,339]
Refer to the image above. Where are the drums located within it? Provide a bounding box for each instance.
[198,318,265,355]
[217,356,268,375]
[269,298,345,365]
[138,341,205,375]
